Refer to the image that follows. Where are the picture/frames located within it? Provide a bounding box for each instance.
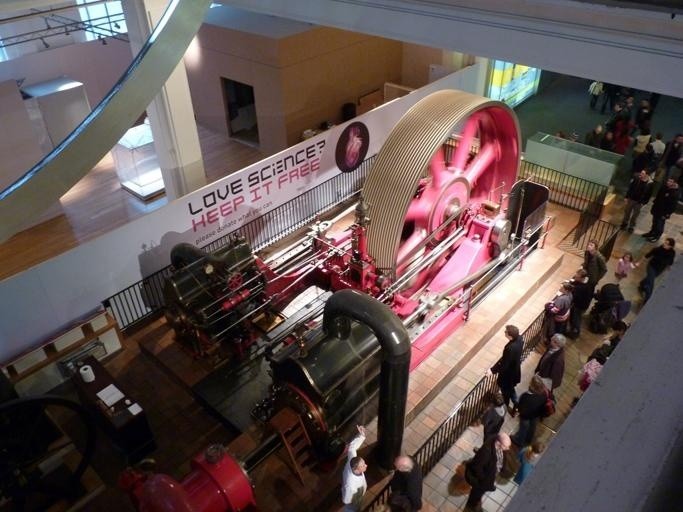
[74,353,153,458]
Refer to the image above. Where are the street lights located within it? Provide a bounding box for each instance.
[543,390,557,418]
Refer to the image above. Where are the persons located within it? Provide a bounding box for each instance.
[474,392,507,452]
[387,456,422,512]
[574,348,610,405]
[585,82,682,183]
[341,425,368,512]
[485,325,524,416]
[555,132,561,137]
[535,334,567,390]
[464,432,499,511]
[637,238,676,303]
[588,319,627,364]
[641,177,683,243]
[570,270,595,339]
[615,252,639,282]
[620,168,660,233]
[512,375,555,433]
[387,493,411,512]
[583,239,608,285]
[541,280,574,346]
[500,420,544,484]
